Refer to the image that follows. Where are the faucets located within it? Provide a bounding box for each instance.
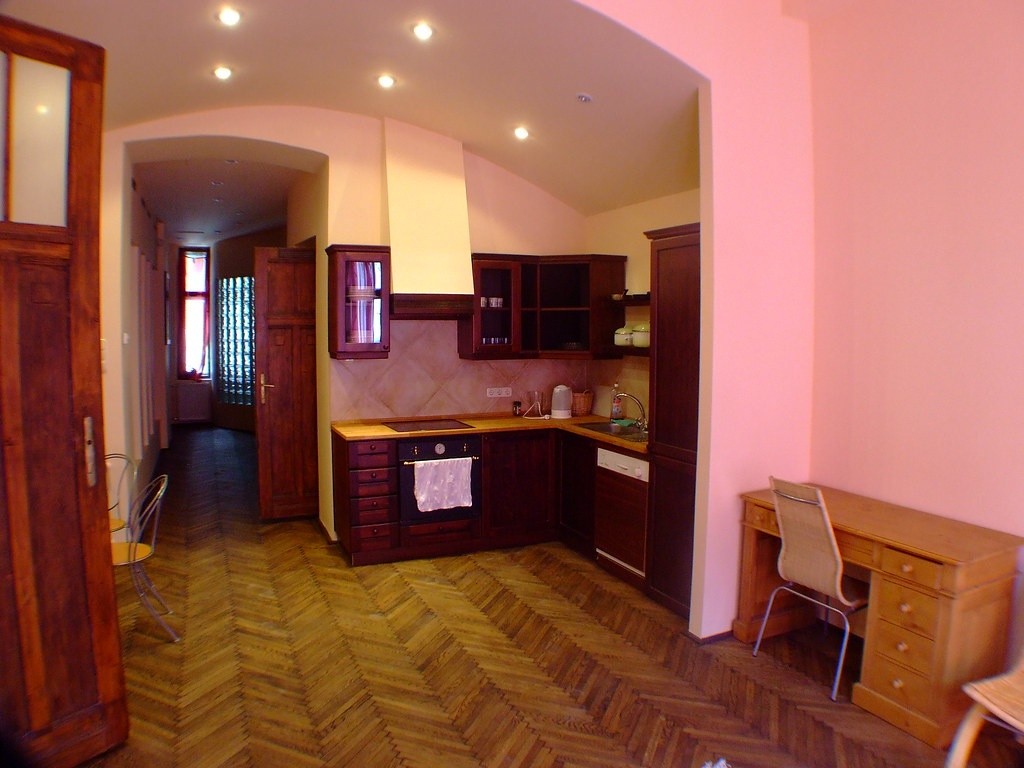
[616,392,647,431]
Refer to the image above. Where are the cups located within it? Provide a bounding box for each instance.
[632,322,650,347]
[529,390,547,416]
[614,328,633,346]
[481,297,503,307]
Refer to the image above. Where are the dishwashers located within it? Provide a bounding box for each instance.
[593,448,649,584]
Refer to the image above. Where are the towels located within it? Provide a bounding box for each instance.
[414,457,473,512]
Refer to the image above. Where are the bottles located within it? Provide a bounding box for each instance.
[610,384,624,419]
[513,401,521,416]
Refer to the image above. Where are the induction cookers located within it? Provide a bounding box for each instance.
[381,418,476,432]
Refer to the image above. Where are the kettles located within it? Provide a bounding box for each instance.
[551,385,573,419]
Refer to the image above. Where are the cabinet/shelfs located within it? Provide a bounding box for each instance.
[325,244,391,361]
[732,483,1023,753]
[332,429,558,567]
[558,222,700,621]
[457,251,627,361]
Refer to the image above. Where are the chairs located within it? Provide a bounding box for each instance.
[752,476,870,701]
[944,657,1024,768]
[104,454,184,646]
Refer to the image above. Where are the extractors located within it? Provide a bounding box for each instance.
[388,293,473,320]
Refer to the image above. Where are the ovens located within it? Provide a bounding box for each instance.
[398,433,484,549]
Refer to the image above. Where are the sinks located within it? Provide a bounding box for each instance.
[578,421,648,441]
